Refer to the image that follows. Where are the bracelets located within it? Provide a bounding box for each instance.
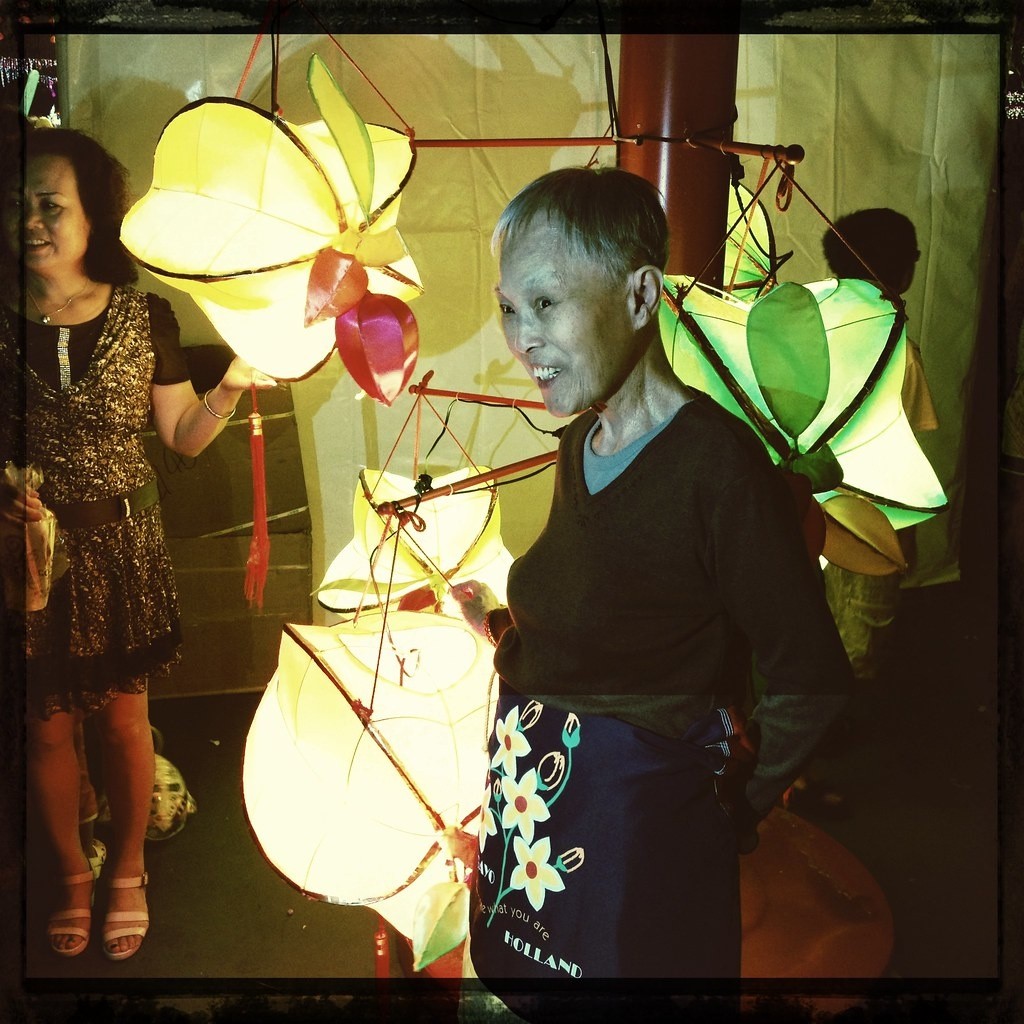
[204,388,237,419]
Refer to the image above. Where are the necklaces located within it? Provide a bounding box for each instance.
[26,276,90,323]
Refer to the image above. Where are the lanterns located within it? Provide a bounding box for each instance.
[118,52,950,1024]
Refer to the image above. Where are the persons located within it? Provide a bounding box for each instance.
[0,128,278,960]
[782,209,942,823]
[467,166,851,1024]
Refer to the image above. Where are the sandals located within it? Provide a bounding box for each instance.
[101,873,151,961]
[43,872,96,956]
